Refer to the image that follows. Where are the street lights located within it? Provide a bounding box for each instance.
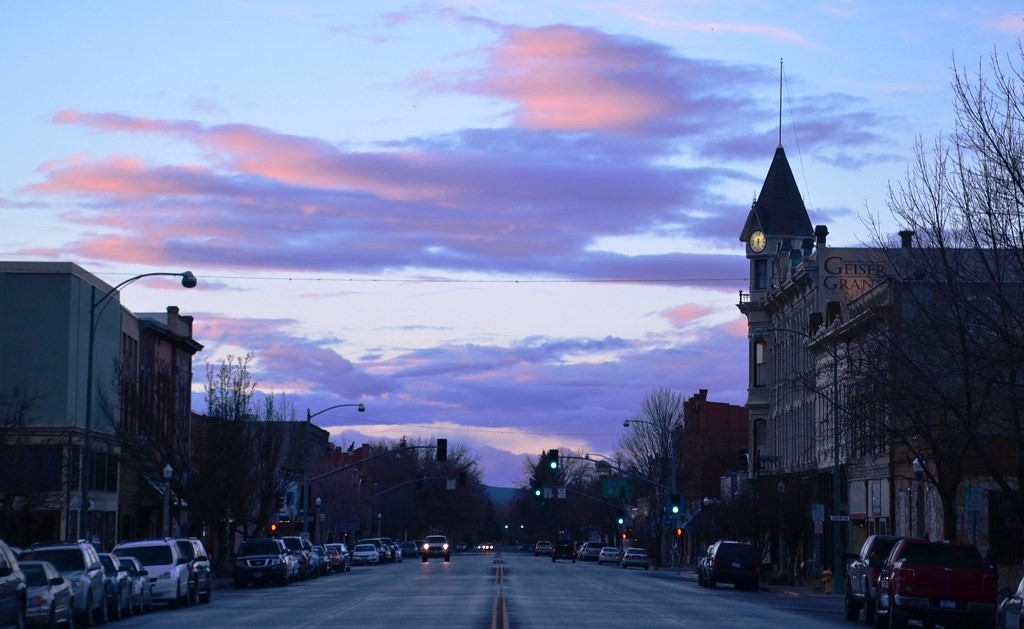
[305,402,365,542]
[585,453,622,532]
[623,420,679,571]
[913,455,927,537]
[78,270,197,538]
[748,326,841,593]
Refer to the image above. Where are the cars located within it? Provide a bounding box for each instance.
[0,535,495,629]
[997,576,1024,629]
[533,541,761,593]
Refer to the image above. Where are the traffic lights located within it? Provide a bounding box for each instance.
[622,533,627,539]
[534,485,542,500]
[504,525,509,529]
[620,486,624,498]
[677,528,683,536]
[618,518,624,524]
[550,449,558,469]
[671,496,680,514]
[521,525,524,528]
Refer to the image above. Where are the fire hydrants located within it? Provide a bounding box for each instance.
[822,570,834,594]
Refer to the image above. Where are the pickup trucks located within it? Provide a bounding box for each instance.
[864,538,999,629]
[844,534,906,623]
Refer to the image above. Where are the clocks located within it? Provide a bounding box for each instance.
[749,231,767,253]
